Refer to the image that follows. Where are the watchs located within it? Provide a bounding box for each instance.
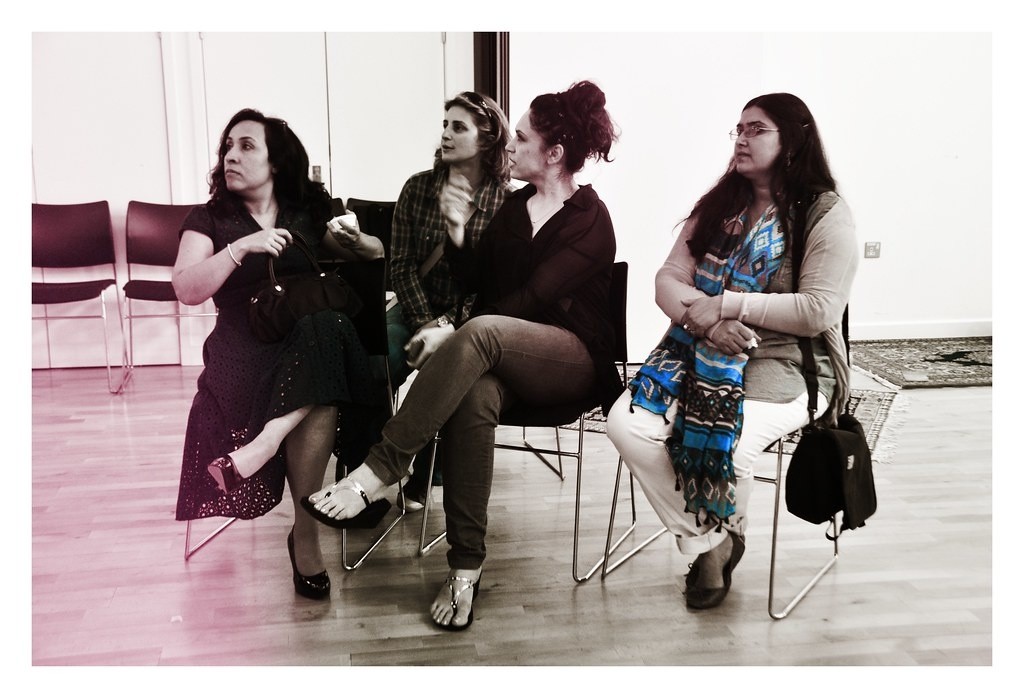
[435,316,450,328]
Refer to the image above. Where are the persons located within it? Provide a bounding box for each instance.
[332,90,522,511]
[601,92,862,609]
[169,107,384,601]
[299,77,615,633]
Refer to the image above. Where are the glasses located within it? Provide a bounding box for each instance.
[730,124,781,140]
[461,91,495,135]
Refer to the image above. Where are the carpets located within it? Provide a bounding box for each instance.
[562,366,908,464]
[846,335,992,391]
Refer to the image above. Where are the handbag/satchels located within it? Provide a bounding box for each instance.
[250,230,350,344]
[785,413,877,540]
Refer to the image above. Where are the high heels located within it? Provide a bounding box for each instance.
[208,456,245,496]
[286,523,330,600]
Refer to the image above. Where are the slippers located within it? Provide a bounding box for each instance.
[431,566,481,632]
[300,475,393,529]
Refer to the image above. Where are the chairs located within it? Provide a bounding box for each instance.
[601,306,851,620]
[419,260,638,582]
[121,198,219,395]
[31,200,129,395]
[184,257,406,571]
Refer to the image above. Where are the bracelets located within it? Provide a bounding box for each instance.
[227,243,242,268]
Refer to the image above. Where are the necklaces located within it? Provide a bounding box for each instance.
[721,200,777,292]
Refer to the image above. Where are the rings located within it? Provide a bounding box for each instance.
[683,322,690,330]
[689,327,696,335]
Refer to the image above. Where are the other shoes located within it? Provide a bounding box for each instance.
[684,530,746,612]
[397,473,433,512]
